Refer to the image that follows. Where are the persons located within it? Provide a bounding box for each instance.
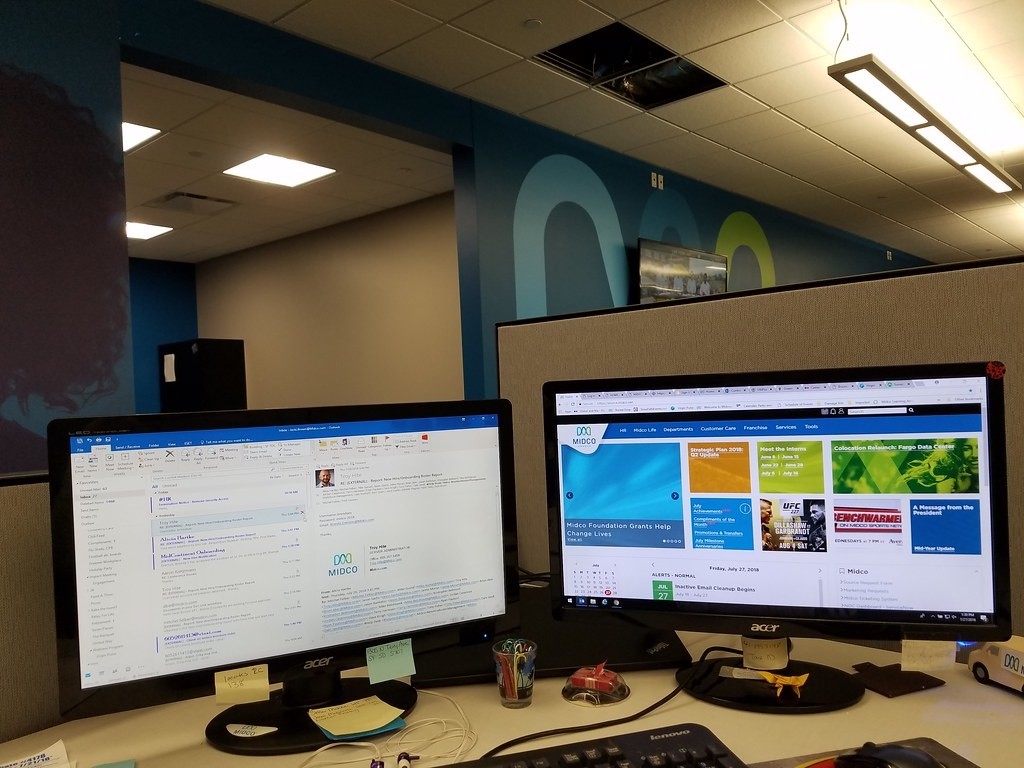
[316,471,335,487]
[760,499,827,552]
[654,269,713,295]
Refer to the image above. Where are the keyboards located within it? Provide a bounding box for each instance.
[434,723,751,768]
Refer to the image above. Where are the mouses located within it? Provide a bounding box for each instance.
[834,741,947,768]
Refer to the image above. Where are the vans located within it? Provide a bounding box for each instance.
[968,635,1024,694]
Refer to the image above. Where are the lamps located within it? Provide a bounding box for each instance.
[827,0,1022,195]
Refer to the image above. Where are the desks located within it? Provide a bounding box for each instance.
[0,630,1024,768]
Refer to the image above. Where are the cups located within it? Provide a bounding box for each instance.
[492,639,539,709]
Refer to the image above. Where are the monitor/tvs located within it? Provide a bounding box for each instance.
[635,237,728,305]
[542,358,1012,717]
[46,397,518,756]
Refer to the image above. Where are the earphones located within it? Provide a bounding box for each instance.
[370,759,384,768]
[397,752,420,768]
